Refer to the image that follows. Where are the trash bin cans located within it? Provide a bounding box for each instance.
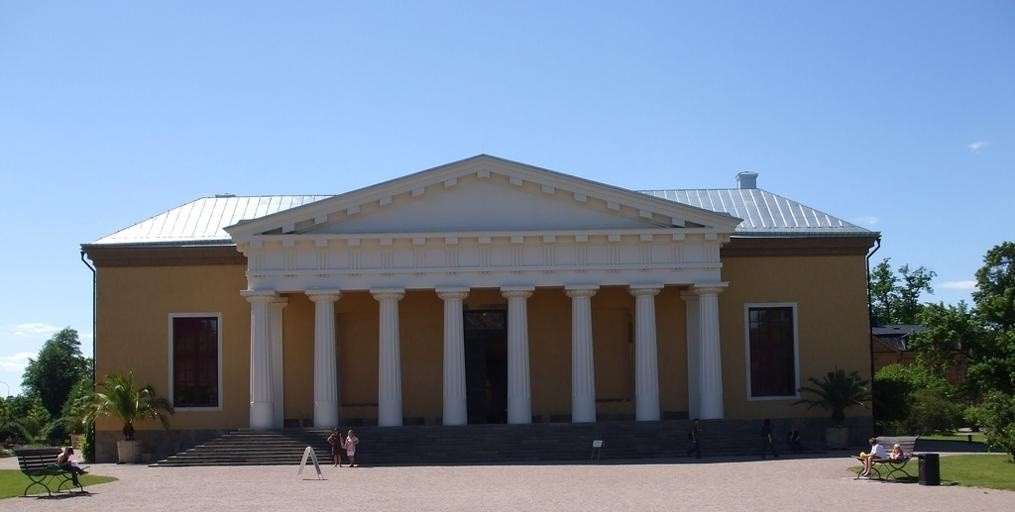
[918,454,940,486]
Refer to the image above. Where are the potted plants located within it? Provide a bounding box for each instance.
[790,365,879,451]
[81,368,176,464]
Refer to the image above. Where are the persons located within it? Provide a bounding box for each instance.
[789,425,802,452]
[687,418,703,458]
[327,426,345,467]
[343,430,359,467]
[862,437,887,476]
[56,448,88,486]
[761,418,780,459]
[889,443,905,461]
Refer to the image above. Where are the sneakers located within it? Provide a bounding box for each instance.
[73,483,79,488]
[79,471,89,475]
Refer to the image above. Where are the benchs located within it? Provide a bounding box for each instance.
[853,435,919,483]
[14,448,90,499]
